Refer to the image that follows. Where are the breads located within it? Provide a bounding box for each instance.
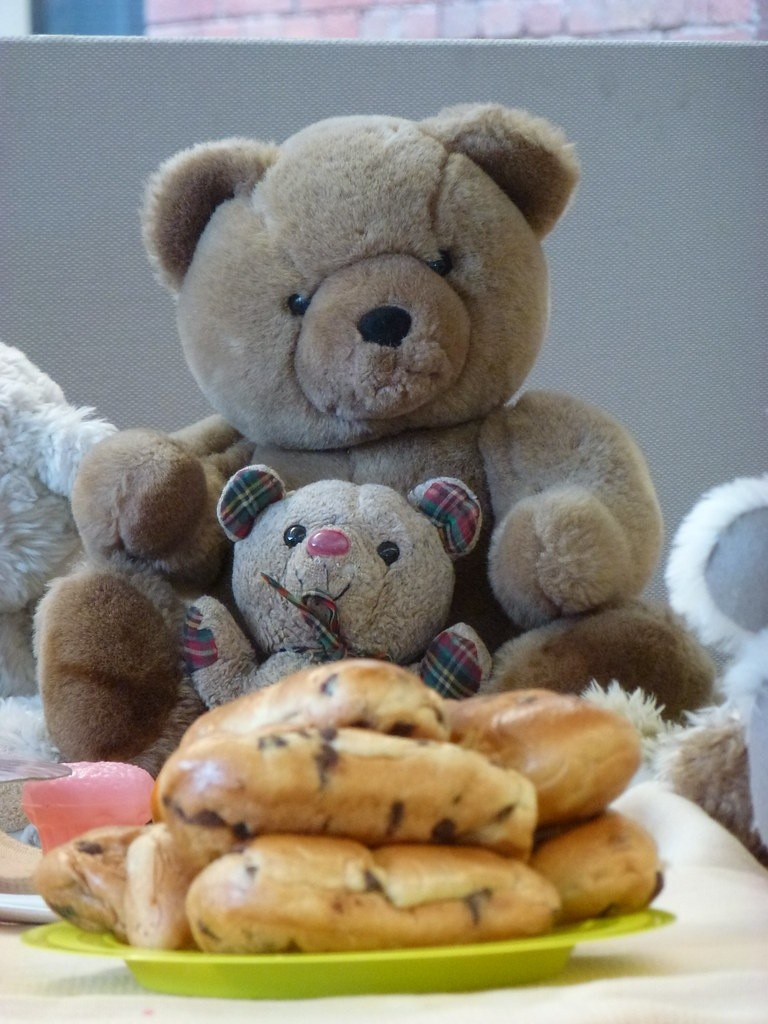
[34,659,660,953]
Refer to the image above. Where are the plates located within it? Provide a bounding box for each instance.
[25,911,679,998]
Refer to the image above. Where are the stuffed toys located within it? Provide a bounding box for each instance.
[187,463,494,707]
[582,467,768,861]
[32,96,728,763]
[1,347,118,699]
